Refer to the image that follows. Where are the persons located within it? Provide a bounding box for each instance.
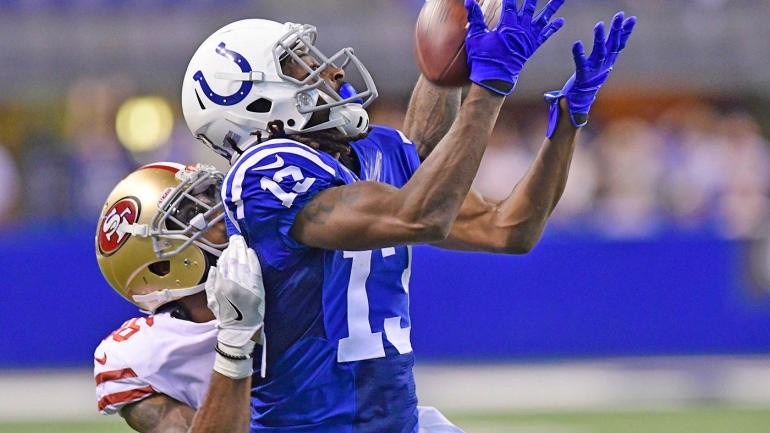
[181,1,634,431]
[91,160,466,432]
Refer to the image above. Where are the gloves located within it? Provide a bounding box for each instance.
[205,234,263,379]
[543,11,638,139]
[464,0,563,96]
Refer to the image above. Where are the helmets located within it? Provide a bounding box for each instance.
[93,162,232,317]
[181,18,380,160]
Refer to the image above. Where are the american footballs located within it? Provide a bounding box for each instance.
[411,0,507,88]
